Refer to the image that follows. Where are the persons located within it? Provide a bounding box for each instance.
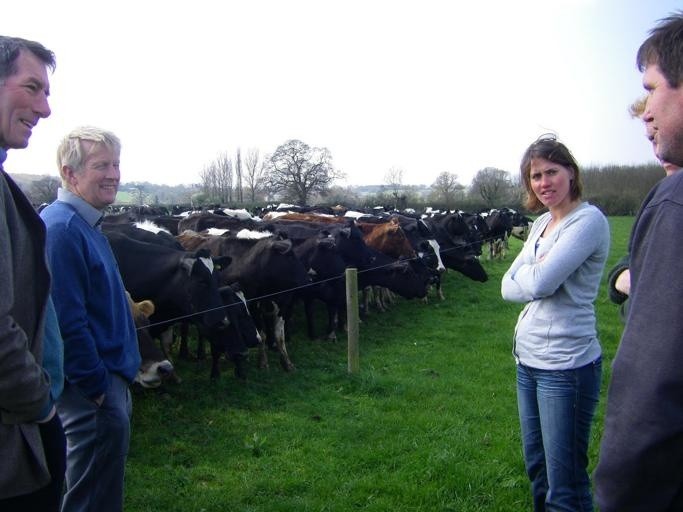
[500,133,611,512]
[607,95,683,326]
[594,9,683,512]
[0,36,142,512]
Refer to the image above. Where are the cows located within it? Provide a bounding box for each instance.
[103,203,535,389]
